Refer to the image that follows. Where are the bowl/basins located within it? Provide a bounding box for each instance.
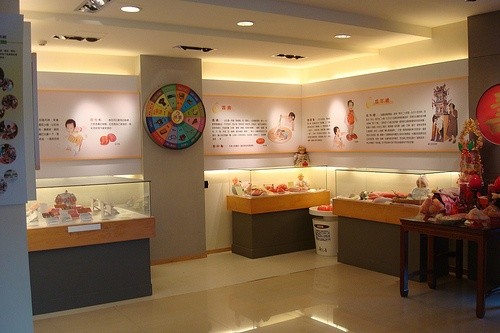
[477,195,488,209]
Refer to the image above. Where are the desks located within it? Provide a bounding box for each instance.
[400,217,500,319]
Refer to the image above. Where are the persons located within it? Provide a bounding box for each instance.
[295,174,310,190]
[411,176,431,199]
[294,147,310,166]
